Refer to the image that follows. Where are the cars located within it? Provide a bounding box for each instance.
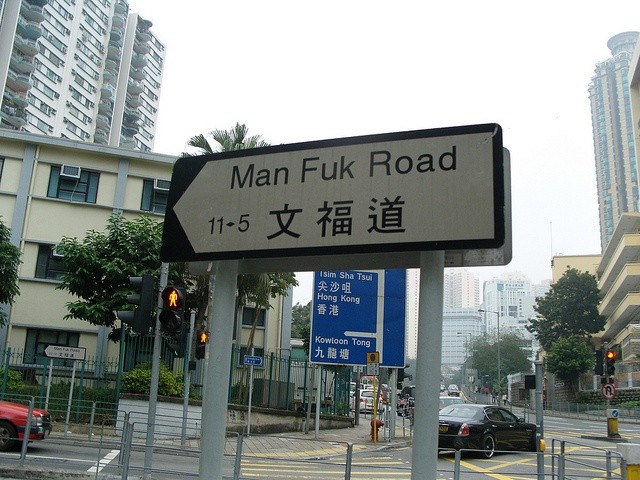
[447,384,460,396]
[439,396,465,411]
[348,382,385,413]
[0,400,53,452]
[397,385,415,417]
[438,403,537,459]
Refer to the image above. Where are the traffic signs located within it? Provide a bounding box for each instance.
[602,383,616,400]
[158,122,506,262]
[308,268,409,369]
[367,363,380,376]
[44,345,86,361]
[243,354,263,366]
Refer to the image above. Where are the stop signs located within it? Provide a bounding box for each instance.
[366,351,379,363]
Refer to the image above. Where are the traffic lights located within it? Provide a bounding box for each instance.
[158,285,186,334]
[606,349,615,376]
[196,329,207,360]
[113,275,154,333]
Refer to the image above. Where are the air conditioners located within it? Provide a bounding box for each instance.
[153,178,171,192]
[53,245,65,257]
[60,163,81,180]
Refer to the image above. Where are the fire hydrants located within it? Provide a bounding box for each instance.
[370,419,384,440]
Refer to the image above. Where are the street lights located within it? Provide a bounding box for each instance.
[477,308,501,407]
[457,332,472,386]
[457,335,468,385]
